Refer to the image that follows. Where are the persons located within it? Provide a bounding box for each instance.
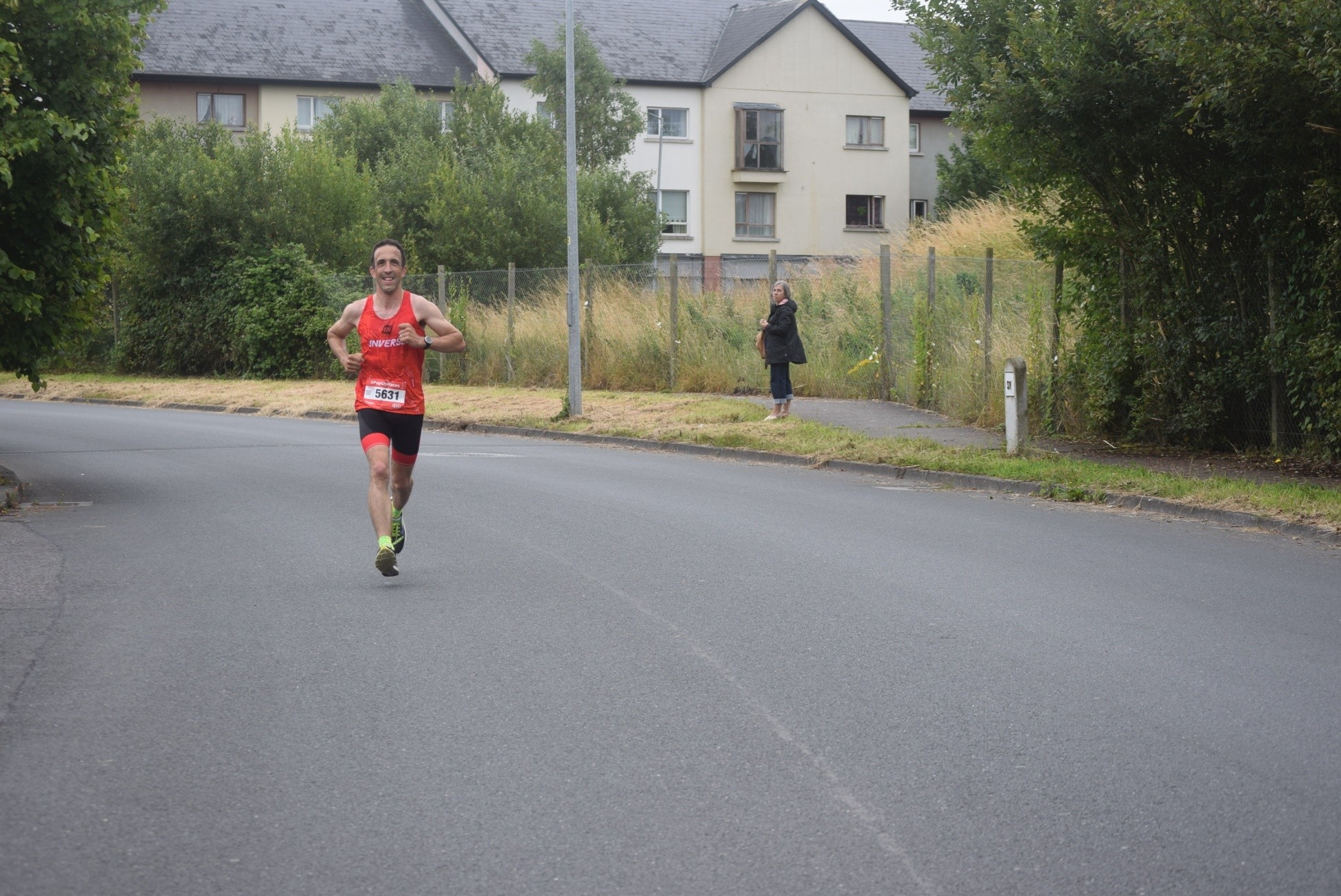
[759,280,807,420]
[327,239,466,577]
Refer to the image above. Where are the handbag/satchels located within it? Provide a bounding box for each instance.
[754,327,765,360]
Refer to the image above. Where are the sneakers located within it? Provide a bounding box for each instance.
[389,497,406,553]
[375,544,399,577]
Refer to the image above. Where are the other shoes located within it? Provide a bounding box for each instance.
[764,412,781,421]
[781,410,789,418]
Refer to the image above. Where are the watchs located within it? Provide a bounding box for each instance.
[423,336,432,350]
